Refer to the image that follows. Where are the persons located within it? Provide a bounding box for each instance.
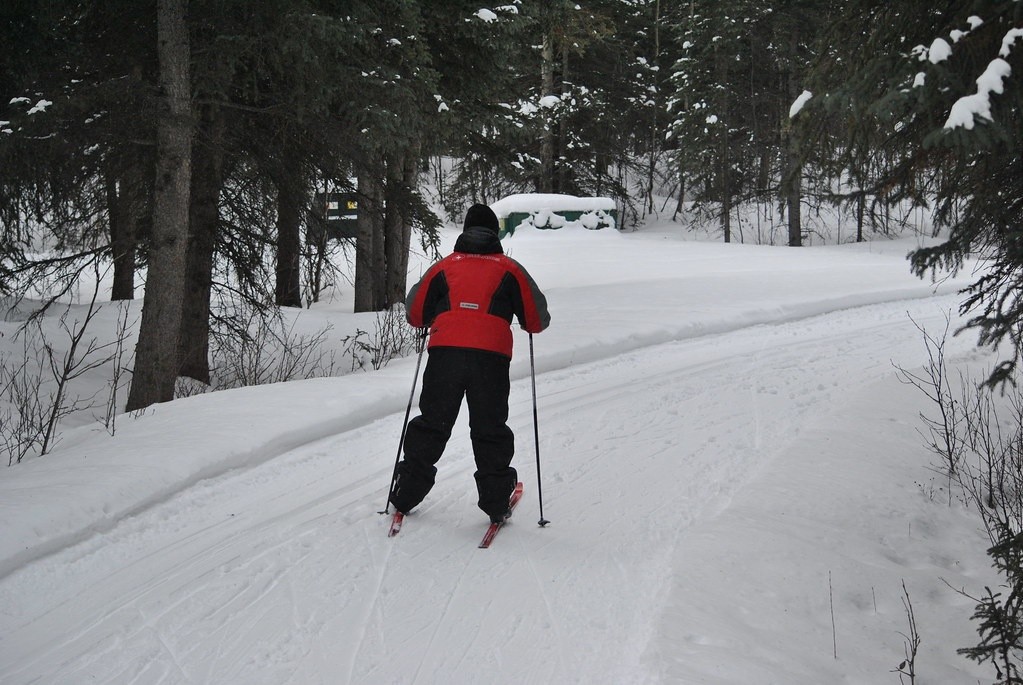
[391,204,551,524]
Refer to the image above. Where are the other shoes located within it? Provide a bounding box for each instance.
[490,506,512,524]
[390,492,406,515]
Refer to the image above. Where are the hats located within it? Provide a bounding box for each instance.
[463,204,499,234]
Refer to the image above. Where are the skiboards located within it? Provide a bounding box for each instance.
[387,481,524,549]
